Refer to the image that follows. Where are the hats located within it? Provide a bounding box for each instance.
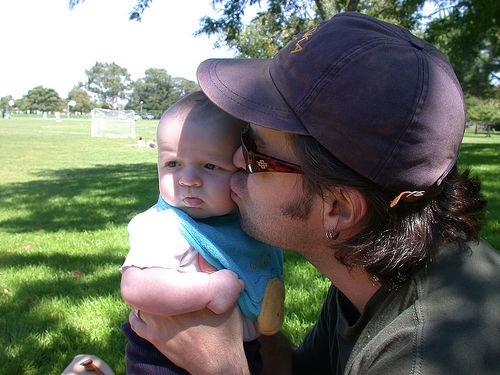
[196,12,466,208]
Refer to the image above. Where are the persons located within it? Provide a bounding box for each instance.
[129,11,500,375]
[137,136,157,149]
[118,92,286,375]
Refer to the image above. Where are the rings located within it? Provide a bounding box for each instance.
[135,309,142,320]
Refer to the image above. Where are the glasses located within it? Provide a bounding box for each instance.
[241,133,303,174]
